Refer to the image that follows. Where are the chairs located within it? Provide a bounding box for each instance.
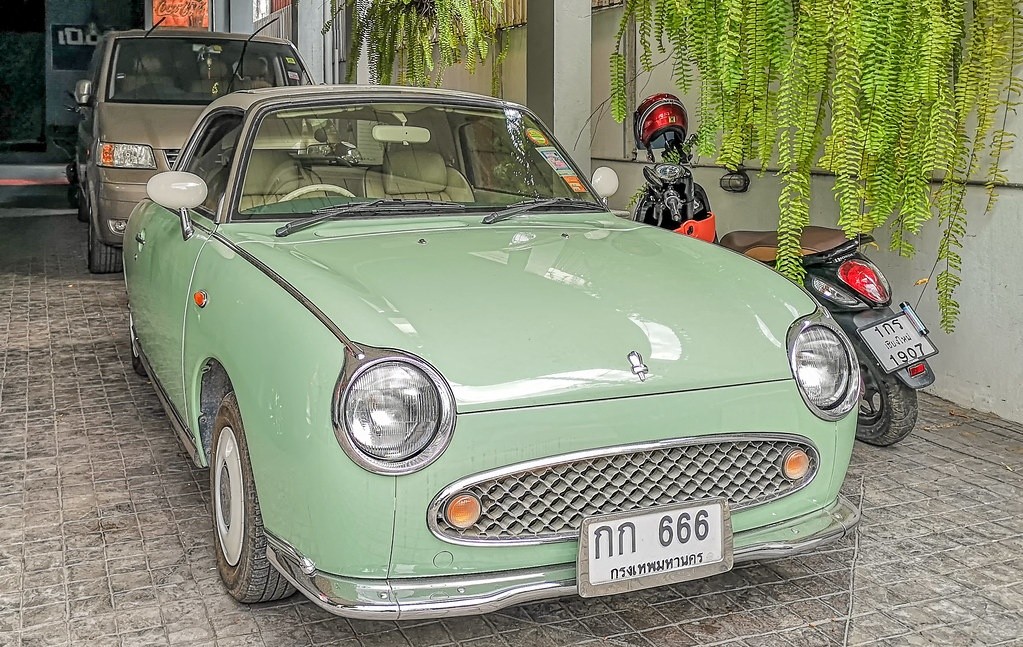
[240,150,323,211]
[244,56,268,88]
[138,52,174,90]
[363,150,476,202]
[192,58,227,92]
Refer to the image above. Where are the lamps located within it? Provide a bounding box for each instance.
[719,170,750,193]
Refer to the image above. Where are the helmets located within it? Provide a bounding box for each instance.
[633,93,689,150]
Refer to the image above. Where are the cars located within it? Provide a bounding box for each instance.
[122,85,862,620]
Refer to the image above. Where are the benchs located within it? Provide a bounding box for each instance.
[309,166,365,201]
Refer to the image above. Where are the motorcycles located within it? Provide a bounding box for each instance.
[631,131,939,446]
[63,90,77,185]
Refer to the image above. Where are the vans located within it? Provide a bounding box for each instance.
[75,16,319,273]
